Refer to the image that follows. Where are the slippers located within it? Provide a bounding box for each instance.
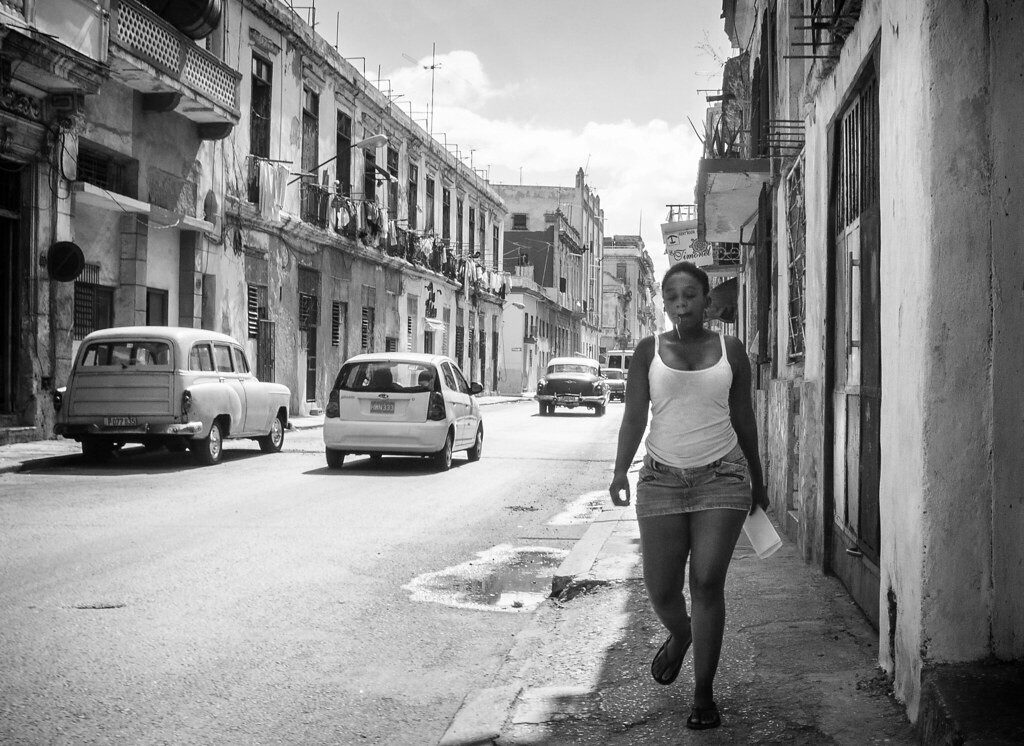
[652,615,694,685]
[686,702,721,729]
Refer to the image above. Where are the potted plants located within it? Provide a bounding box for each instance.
[686,113,744,157]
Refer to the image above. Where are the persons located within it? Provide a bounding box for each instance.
[610,261,770,731]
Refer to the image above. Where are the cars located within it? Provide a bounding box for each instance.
[324,352,484,470]
[532,358,612,418]
[603,368,627,404]
[53,327,291,465]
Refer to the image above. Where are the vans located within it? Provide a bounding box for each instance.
[606,350,637,387]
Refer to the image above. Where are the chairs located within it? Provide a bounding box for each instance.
[368,368,397,388]
